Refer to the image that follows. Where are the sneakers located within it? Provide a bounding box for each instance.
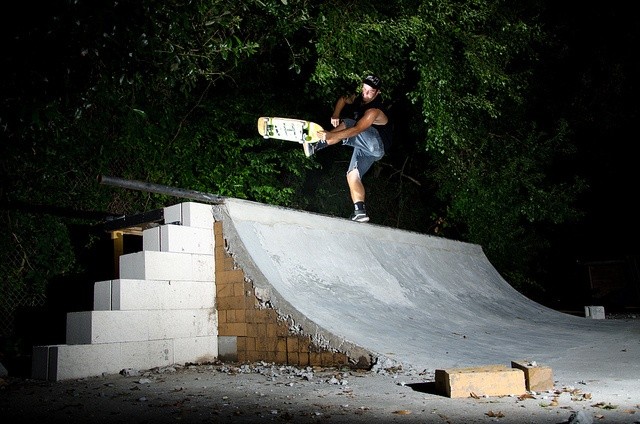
[303,142,315,157]
[349,210,370,222]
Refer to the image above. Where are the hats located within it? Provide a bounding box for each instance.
[363,75,382,89]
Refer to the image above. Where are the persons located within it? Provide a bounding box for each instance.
[304,75,391,223]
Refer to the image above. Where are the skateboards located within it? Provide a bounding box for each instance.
[258,117,324,143]
